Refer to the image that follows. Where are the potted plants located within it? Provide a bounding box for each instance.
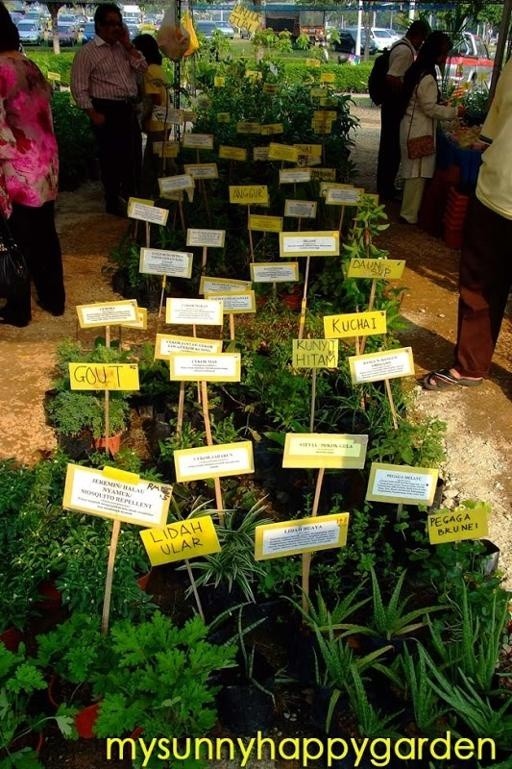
[0,90,512,768]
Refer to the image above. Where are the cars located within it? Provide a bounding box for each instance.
[325,26,494,94]
[11,9,235,46]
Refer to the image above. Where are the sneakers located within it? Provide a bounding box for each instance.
[0,305,32,328]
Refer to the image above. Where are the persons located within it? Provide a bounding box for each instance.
[392,31,466,224]
[0,2,65,326]
[319,44,329,61]
[376,21,428,203]
[70,3,148,218]
[132,35,172,192]
[419,54,512,389]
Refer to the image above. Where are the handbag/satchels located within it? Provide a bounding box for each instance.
[0,206,30,290]
[406,135,437,160]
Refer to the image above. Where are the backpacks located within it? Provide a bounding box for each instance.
[368,43,415,107]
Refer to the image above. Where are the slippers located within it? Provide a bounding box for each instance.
[422,367,484,391]
